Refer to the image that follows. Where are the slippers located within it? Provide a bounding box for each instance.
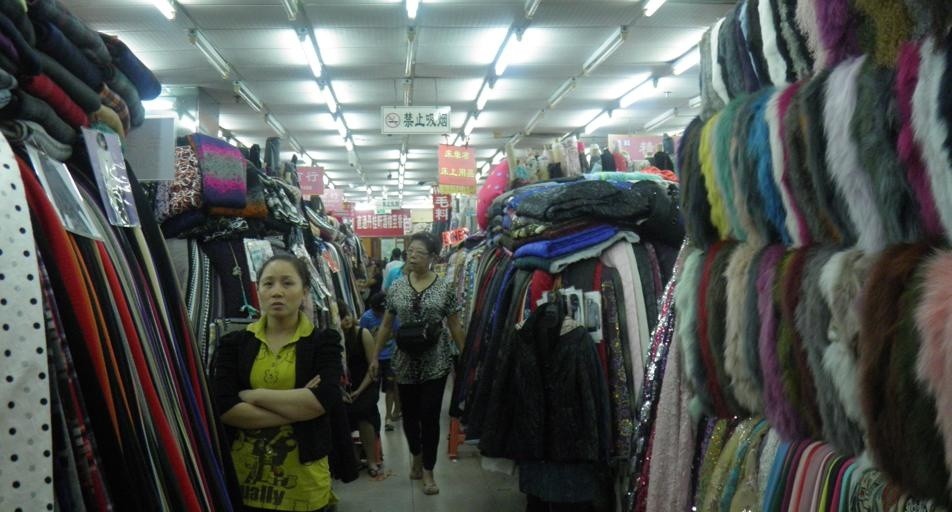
[368,468,381,477]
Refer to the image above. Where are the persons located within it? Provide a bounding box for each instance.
[364,247,407,305]
[337,298,380,479]
[212,256,343,509]
[361,292,401,431]
[368,232,466,496]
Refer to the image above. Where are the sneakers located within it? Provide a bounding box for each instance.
[385,419,394,430]
[410,464,422,479]
[422,481,439,494]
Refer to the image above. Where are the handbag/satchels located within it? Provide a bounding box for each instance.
[396,320,439,353]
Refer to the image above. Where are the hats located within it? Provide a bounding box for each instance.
[371,292,388,310]
